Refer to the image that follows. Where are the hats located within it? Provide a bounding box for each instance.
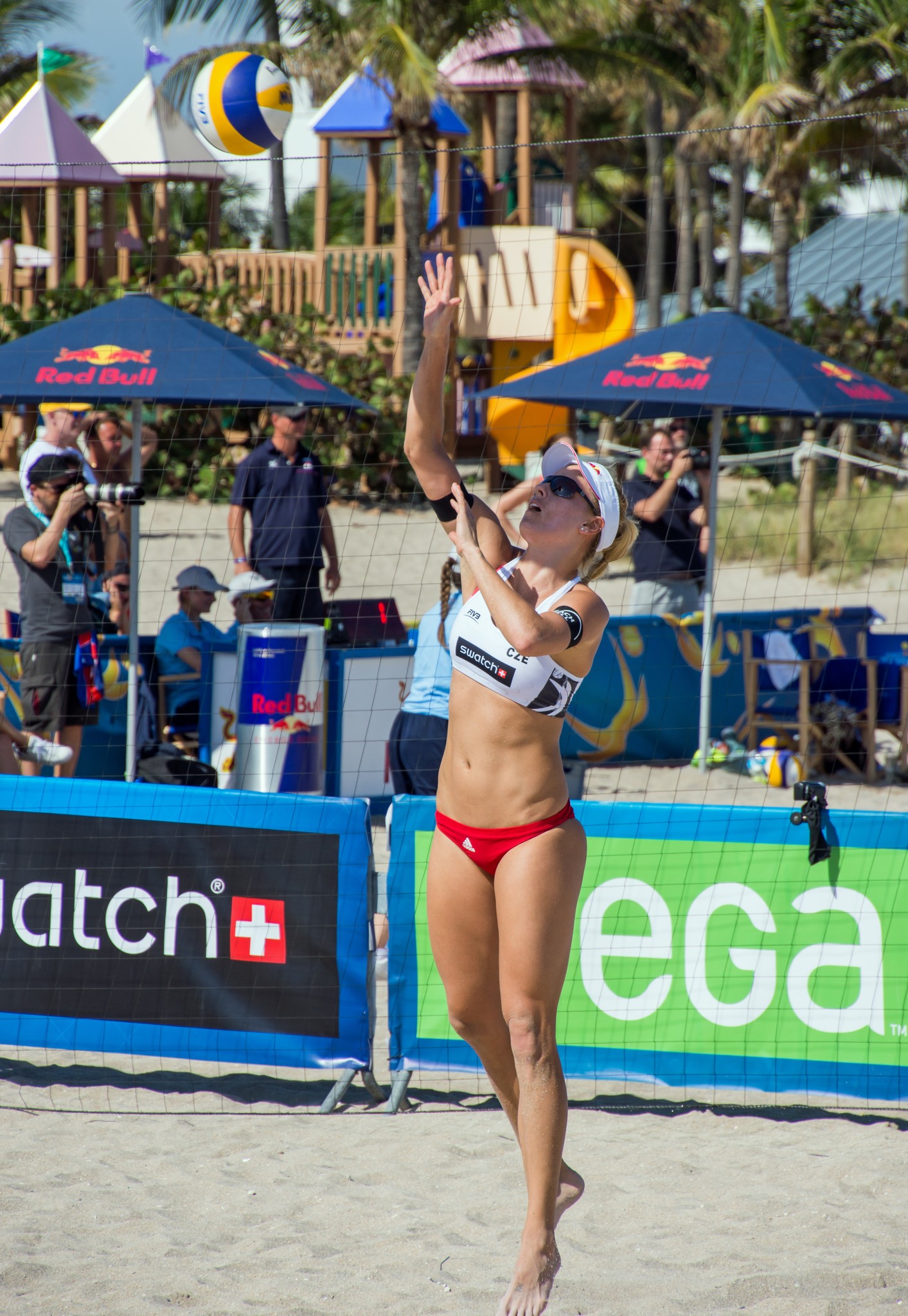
[100,563,130,582]
[653,416,687,430]
[228,572,278,602]
[269,406,310,417]
[171,564,229,593]
[26,454,80,483]
[38,399,93,413]
[541,442,620,553]
[450,547,463,574]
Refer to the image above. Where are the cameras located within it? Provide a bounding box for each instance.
[685,447,711,469]
[67,477,145,505]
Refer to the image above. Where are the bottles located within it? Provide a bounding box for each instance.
[746,750,768,783]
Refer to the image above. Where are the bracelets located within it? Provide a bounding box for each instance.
[235,558,248,563]
[425,476,473,525]
[559,607,584,648]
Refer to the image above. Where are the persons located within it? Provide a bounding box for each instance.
[620,427,710,618]
[228,403,341,622]
[230,575,279,623]
[155,567,230,764]
[390,544,463,798]
[403,253,622,1316]
[0,395,158,779]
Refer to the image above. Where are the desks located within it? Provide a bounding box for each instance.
[196,644,416,802]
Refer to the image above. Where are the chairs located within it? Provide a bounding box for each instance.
[742,628,876,783]
[144,644,199,762]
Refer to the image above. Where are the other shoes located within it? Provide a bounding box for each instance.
[11,730,73,765]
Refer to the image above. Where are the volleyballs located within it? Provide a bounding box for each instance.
[758,735,806,790]
[189,50,294,156]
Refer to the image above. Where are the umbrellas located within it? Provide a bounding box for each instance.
[469,309,908,774]
[0,294,379,782]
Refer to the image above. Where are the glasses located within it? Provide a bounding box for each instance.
[37,476,86,494]
[541,475,600,516]
[113,584,131,592]
[244,591,274,601]
[670,425,689,433]
[53,410,88,419]
[284,413,307,423]
